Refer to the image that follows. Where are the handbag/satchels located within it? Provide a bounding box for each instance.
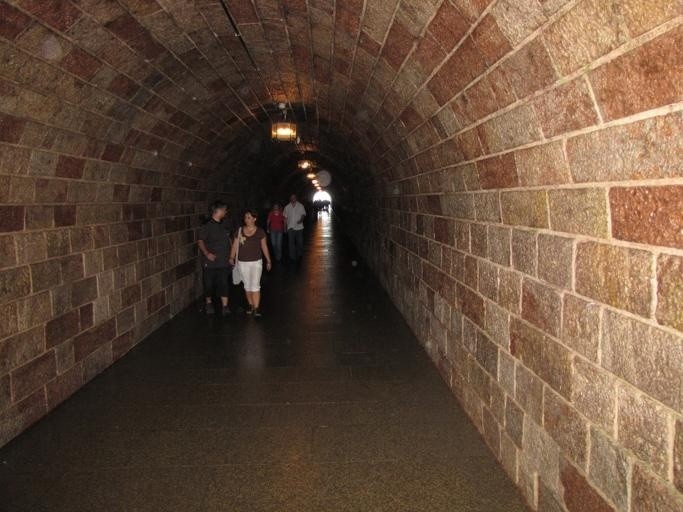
[233,268,242,284]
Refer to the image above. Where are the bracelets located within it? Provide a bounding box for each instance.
[228,257,234,260]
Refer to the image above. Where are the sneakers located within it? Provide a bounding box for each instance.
[247,305,262,316]
[206,303,231,317]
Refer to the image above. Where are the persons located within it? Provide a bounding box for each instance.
[282,194,306,263]
[195,201,234,316]
[266,203,286,261]
[301,198,330,223]
[227,208,271,318]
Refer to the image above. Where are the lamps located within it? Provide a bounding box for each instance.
[269,103,317,172]
